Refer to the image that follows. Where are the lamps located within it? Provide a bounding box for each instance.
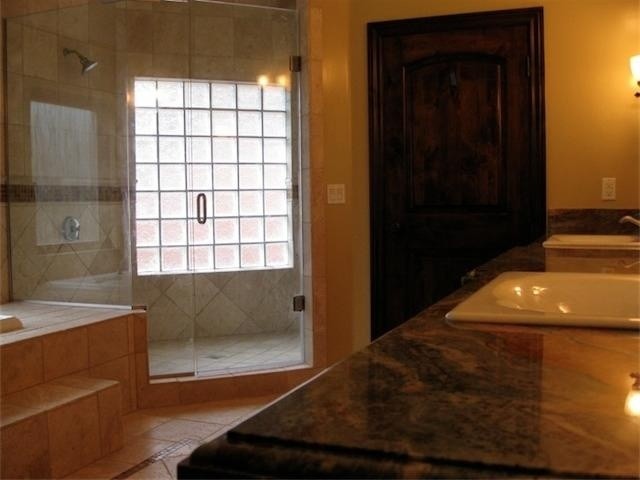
[255,72,290,90]
[630,55,640,97]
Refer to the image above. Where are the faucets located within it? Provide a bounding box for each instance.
[617,215,640,228]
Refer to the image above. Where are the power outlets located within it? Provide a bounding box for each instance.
[602,177,616,201]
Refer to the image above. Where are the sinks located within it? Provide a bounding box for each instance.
[44,269,127,305]
[446,270,640,331]
[0,314,23,333]
[543,233,640,250]
